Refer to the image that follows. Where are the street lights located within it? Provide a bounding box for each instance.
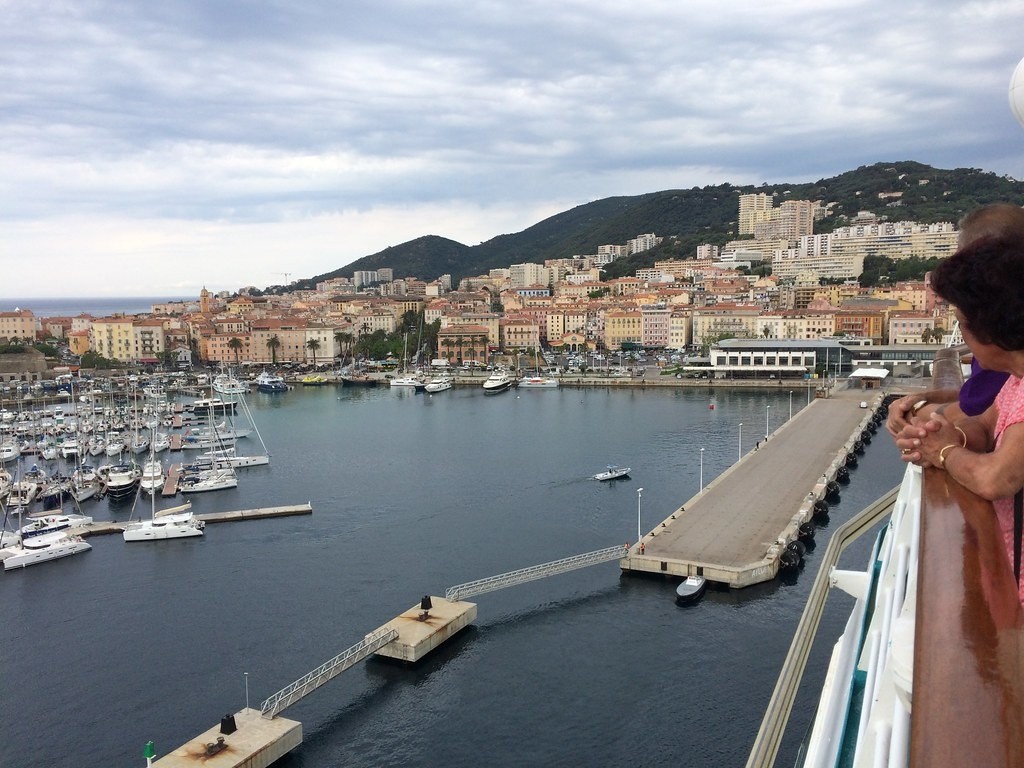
[739,422,744,460]
[637,488,644,543]
[823,369,826,388]
[699,447,704,494]
[766,405,770,438]
[835,363,838,383]
[789,389,793,420]
[807,379,811,405]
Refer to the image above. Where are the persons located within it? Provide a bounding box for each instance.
[885,201,1024,609]
[609,469,612,473]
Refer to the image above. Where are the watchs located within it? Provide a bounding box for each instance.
[911,400,928,417]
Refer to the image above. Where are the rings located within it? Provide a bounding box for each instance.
[901,448,911,453]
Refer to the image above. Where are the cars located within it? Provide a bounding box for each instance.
[544,344,702,371]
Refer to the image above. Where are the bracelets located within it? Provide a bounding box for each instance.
[939,444,961,471]
[955,426,966,448]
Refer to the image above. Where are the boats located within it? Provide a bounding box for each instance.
[595,464,632,481]
[340,334,378,385]
[388,330,426,388]
[301,374,329,386]
[517,376,559,389]
[676,576,706,604]
[482,367,512,396]
[424,378,452,393]
[259,373,290,393]
[0,370,270,571]
